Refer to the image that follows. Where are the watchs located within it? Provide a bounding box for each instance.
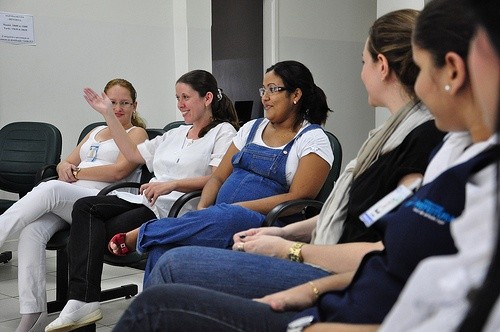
[288,242,305,264]
[73,168,81,181]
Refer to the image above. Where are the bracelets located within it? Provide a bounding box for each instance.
[308,281,319,298]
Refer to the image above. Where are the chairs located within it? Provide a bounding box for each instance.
[0,120,344,312]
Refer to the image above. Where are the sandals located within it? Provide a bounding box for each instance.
[108,233,137,256]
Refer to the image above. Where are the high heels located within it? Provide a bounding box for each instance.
[27,311,47,332]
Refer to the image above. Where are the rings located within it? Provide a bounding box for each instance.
[237,243,245,253]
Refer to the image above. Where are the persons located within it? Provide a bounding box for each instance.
[0,0,500,332]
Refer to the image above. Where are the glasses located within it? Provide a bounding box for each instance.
[258,85,286,97]
[111,99,135,109]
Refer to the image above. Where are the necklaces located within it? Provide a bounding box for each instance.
[177,126,199,163]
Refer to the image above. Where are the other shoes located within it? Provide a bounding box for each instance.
[45,301,103,332]
